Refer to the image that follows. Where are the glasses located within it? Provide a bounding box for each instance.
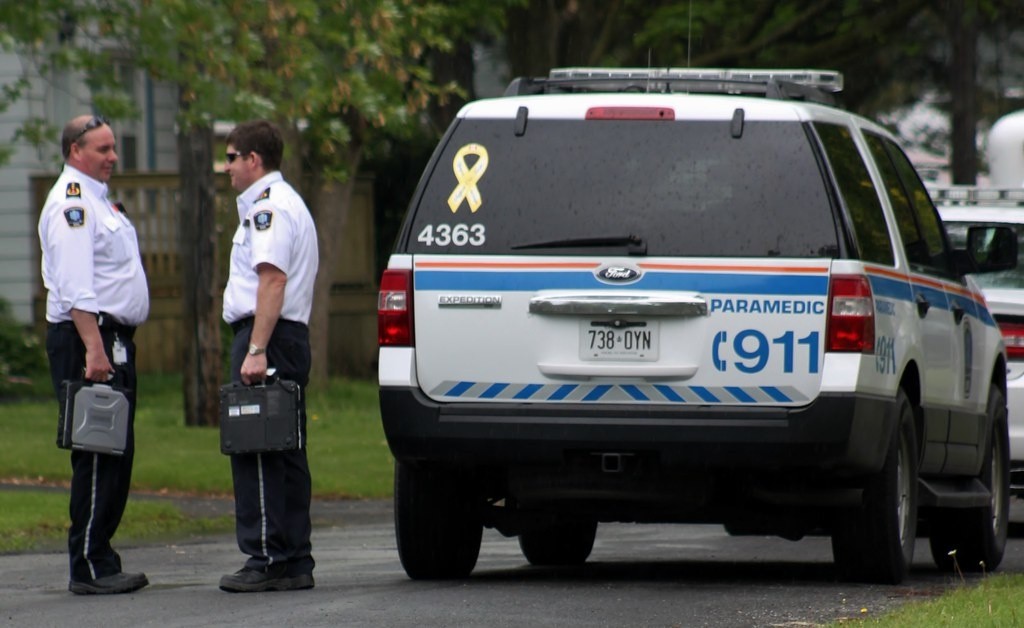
[70,116,110,141]
[226,152,243,162]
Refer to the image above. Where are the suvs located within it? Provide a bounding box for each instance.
[927,186,1023,495]
[376,66,1010,584]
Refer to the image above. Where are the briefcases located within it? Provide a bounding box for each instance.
[220,377,305,457]
[56,371,134,456]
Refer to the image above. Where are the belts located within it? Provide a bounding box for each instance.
[230,315,308,336]
[47,317,137,339]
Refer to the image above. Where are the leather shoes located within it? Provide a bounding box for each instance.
[219,566,315,593]
[68,571,149,595]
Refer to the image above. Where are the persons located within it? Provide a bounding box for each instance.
[220,120,319,592]
[36,114,149,595]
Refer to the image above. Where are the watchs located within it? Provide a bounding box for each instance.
[248,344,266,356]
